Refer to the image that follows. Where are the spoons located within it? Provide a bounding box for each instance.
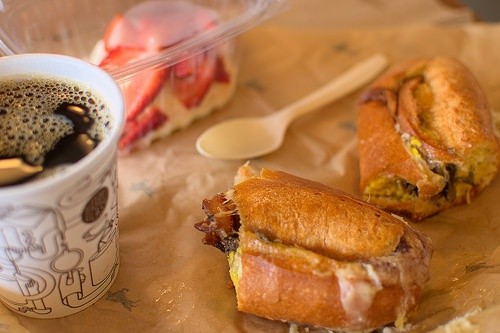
[193,53,390,161]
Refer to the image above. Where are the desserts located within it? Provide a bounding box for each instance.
[87,1,239,152]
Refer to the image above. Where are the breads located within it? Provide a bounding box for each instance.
[357,52,499,221]
[190,163,432,333]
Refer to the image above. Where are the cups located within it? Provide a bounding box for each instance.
[0,52,128,320]
